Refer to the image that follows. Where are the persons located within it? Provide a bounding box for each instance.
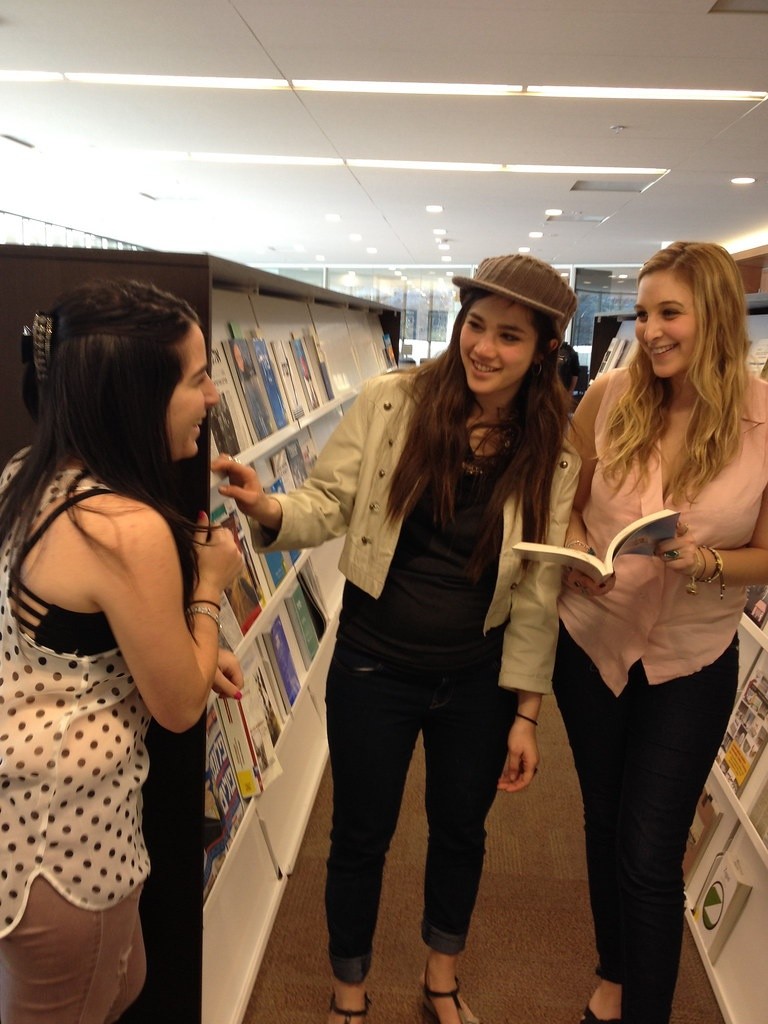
[1,279,245,1023]
[549,240,768,1024]
[213,255,582,1024]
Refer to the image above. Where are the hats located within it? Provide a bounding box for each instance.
[452,254,577,344]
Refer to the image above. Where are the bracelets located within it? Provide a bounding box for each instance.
[185,607,223,632]
[516,712,538,725]
[566,540,597,557]
[686,545,727,599]
[188,599,221,610]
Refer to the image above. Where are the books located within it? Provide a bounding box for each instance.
[202,319,333,906]
[382,332,398,369]
[715,663,767,799]
[512,507,682,587]
[681,782,724,893]
[743,582,768,630]
[748,339,768,381]
[693,851,754,963]
[593,337,626,381]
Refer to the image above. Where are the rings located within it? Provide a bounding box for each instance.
[664,550,679,559]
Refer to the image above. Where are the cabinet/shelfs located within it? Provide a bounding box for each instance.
[0,242,403,1024]
[587,304,768,1024]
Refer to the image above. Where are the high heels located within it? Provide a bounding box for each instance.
[422,959,480,1024]
[327,993,373,1024]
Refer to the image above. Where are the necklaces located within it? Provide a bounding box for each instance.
[462,415,516,474]
